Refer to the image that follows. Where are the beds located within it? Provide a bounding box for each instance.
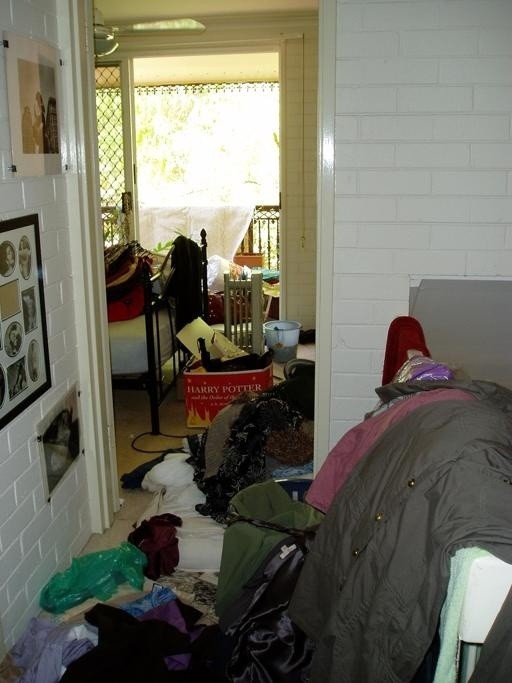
[105,227,210,437]
[410,271,512,683]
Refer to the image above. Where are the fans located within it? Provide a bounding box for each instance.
[93,7,206,40]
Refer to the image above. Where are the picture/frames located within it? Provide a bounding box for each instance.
[4,35,69,177]
[33,380,84,509]
[1,213,53,427]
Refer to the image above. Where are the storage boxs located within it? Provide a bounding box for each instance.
[184,349,274,429]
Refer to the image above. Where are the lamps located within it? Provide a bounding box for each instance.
[93,39,120,57]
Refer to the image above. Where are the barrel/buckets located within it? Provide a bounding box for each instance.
[263,320,302,362]
